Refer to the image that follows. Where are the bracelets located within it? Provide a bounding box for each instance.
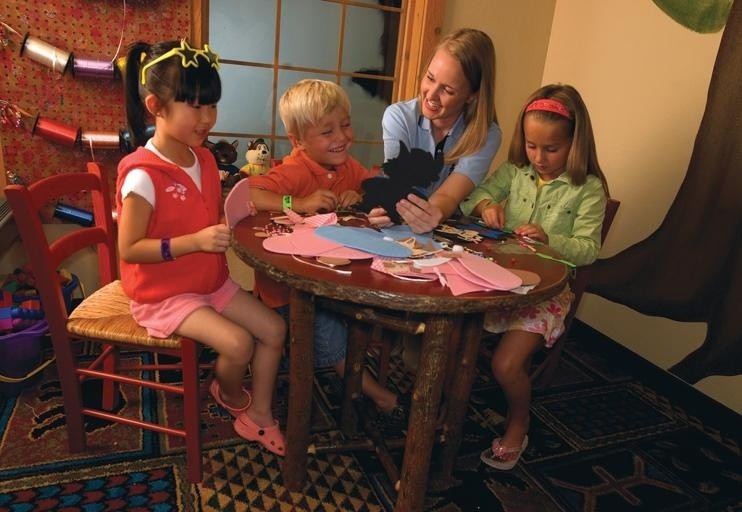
[281,190,294,214]
[158,236,175,263]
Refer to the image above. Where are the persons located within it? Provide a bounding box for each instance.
[247,75,411,424]
[380,26,502,236]
[114,35,290,460]
[458,83,612,473]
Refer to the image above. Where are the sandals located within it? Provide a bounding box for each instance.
[233,411,285,456]
[211,378,251,418]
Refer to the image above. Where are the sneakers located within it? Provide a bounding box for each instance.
[378,392,445,428]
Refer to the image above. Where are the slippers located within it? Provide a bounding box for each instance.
[480,433,528,470]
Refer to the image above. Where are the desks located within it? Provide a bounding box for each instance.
[230,209,570,511]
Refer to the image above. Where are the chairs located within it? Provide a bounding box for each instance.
[4,161,226,483]
[480,200,622,390]
